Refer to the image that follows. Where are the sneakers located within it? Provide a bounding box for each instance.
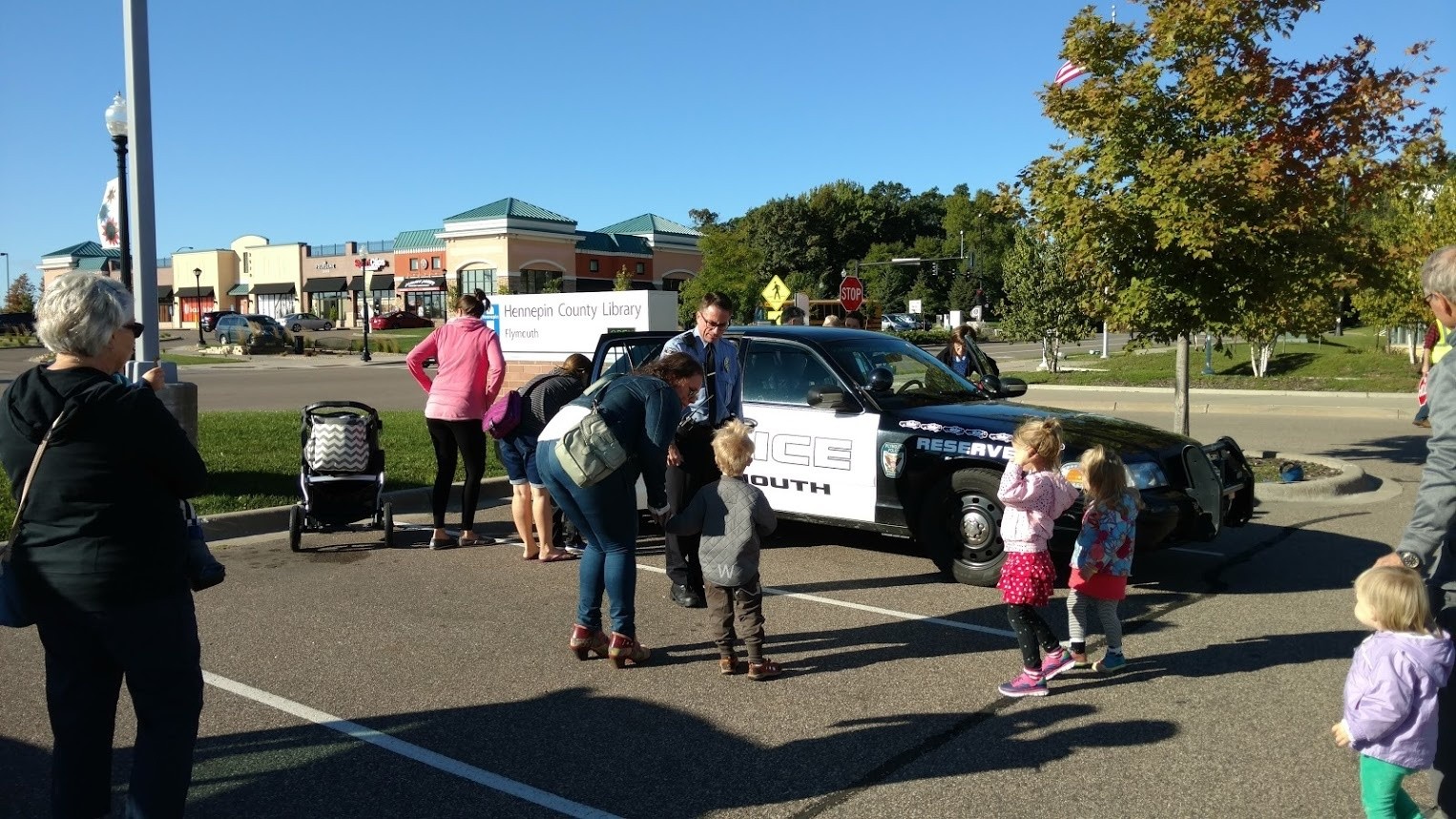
[669,581,700,608]
[1091,652,1127,674]
[1068,651,1090,666]
[1040,647,1078,680]
[429,535,458,549]
[720,656,738,674]
[748,659,782,680]
[997,671,1050,697]
[458,534,496,546]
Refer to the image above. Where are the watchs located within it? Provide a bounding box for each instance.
[1395,548,1424,570]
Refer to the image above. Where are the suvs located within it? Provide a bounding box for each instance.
[201,310,241,333]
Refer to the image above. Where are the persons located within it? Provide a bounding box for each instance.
[405,287,507,551]
[532,350,705,672]
[1373,240,1455,819]
[113,364,168,393]
[778,306,810,385]
[1413,318,1454,428]
[659,288,745,609]
[1330,565,1455,819]
[844,310,865,329]
[493,353,594,564]
[660,414,783,682]
[925,325,1002,394]
[549,495,588,551]
[1065,444,1146,673]
[822,314,841,327]
[994,413,1080,698]
[2,267,210,818]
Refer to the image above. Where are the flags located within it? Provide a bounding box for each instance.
[97,176,121,251]
[1052,53,1096,86]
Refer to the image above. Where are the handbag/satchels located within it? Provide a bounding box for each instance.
[483,389,524,440]
[554,412,629,488]
[0,530,36,630]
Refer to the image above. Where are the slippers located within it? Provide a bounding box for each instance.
[543,549,576,562]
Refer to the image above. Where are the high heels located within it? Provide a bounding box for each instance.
[570,624,608,661]
[607,632,649,670]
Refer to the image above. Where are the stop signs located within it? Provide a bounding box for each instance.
[839,276,863,312]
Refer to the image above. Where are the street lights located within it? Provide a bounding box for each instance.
[106,93,132,296]
[193,268,207,347]
[0,252,12,311]
[358,244,373,361]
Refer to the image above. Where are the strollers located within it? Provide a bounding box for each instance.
[289,400,396,551]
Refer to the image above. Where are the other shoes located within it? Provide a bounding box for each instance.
[1412,419,1431,428]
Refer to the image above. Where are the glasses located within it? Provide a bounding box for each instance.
[1422,294,1435,308]
[687,388,700,399]
[700,313,730,331]
[123,321,145,338]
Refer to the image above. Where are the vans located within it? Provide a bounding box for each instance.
[215,315,285,345]
[881,313,932,331]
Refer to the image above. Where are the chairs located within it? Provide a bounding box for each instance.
[744,348,862,415]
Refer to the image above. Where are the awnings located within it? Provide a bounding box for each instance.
[172,286,214,297]
[346,274,394,291]
[248,283,296,295]
[158,285,173,300]
[397,276,447,292]
[302,277,347,293]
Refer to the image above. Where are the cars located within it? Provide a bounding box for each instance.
[371,311,431,331]
[274,312,334,332]
[587,326,1252,594]
